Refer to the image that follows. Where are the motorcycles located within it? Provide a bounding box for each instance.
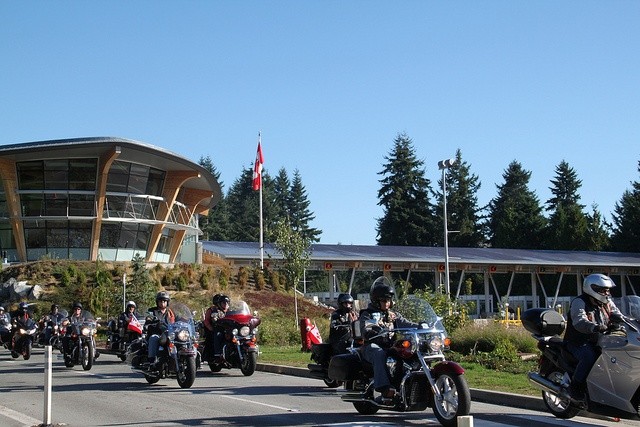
[519,297,640,420]
[307,342,362,388]
[196,301,262,376]
[59,311,102,370]
[328,297,471,427]
[124,303,202,389]
[8,317,39,359]
[105,312,147,361]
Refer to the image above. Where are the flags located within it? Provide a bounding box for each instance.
[252,141,264,190]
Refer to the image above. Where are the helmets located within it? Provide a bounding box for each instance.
[72,301,82,310]
[18,302,29,313]
[51,304,58,310]
[370,276,396,307]
[126,301,137,309]
[213,294,230,304]
[337,294,354,305]
[156,291,170,306]
[583,274,616,305]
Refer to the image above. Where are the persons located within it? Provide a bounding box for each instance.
[11,301,37,343]
[204,293,236,364]
[64,302,101,356]
[45,303,67,336]
[353,275,434,404]
[144,290,190,367]
[120,300,137,346]
[562,273,633,407]
[328,292,357,355]
[1,304,12,339]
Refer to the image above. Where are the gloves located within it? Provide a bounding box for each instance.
[594,325,610,335]
